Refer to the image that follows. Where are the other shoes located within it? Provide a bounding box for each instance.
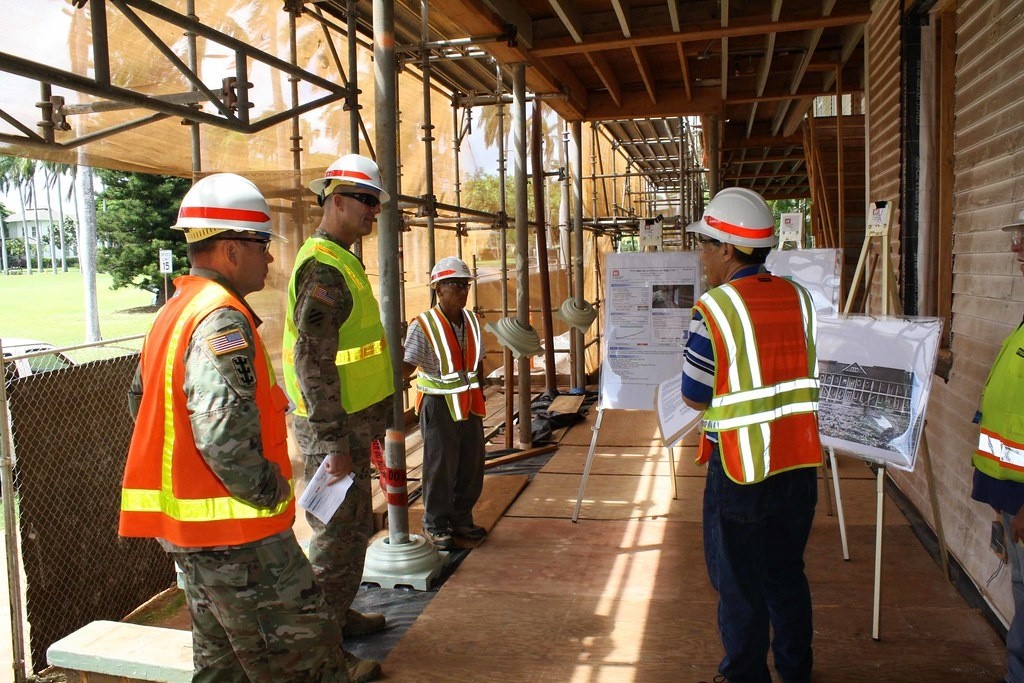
[449,523,488,544]
[425,529,449,550]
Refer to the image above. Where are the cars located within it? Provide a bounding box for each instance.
[0,338,83,497]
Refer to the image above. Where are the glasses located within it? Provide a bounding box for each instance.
[336,189,381,209]
[440,281,473,290]
[1012,231,1024,243]
[697,233,719,247]
[214,235,272,254]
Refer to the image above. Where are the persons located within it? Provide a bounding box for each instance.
[680,186,824,682]
[970,211,1024,683]
[283,154,395,683]
[400,256,488,550]
[115,173,351,683]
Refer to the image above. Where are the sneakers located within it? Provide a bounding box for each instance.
[343,609,386,638]
[343,650,381,682]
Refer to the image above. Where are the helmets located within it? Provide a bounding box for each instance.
[1003,209,1024,229]
[428,259,472,287]
[308,153,383,197]
[169,172,274,233]
[686,186,778,248]
